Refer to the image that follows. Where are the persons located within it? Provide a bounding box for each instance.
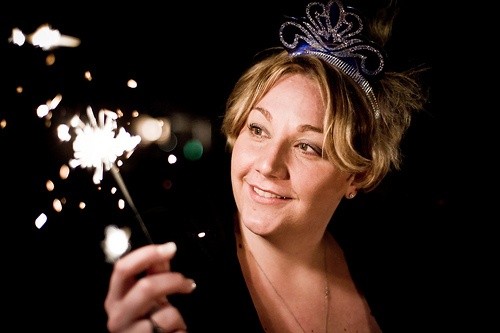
[103,1,433,332]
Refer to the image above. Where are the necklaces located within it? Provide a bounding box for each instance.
[239,233,330,333]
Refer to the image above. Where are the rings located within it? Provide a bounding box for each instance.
[148,317,162,333]
